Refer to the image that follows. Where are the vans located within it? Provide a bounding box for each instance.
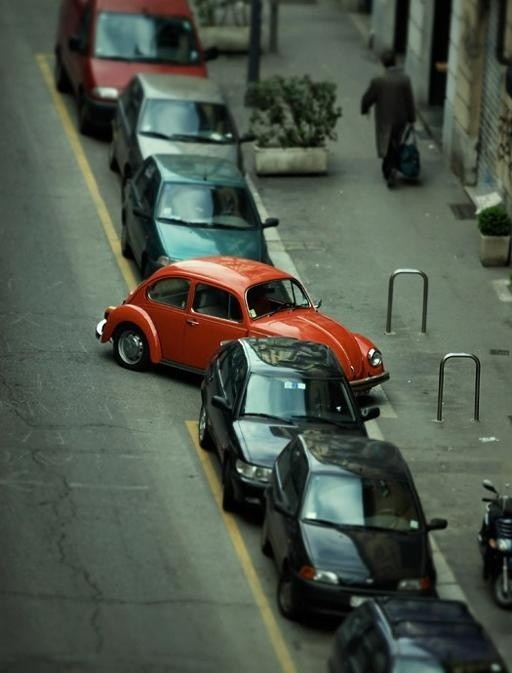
[54,0,220,136]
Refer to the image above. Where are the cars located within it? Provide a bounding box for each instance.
[119,153,281,269]
[198,336,380,524]
[260,433,449,627]
[109,73,259,176]
[95,256,391,399]
[333,596,510,671]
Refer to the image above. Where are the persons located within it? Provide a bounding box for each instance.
[359,48,416,189]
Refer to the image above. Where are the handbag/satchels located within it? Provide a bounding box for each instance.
[397,143,420,177]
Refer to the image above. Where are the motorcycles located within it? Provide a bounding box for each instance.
[476,479,512,607]
